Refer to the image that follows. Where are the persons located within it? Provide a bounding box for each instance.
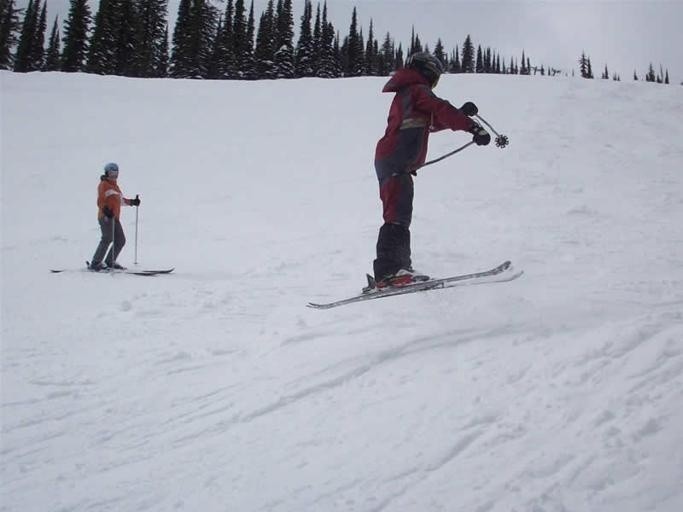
[374,52,491,288]
[88,163,140,272]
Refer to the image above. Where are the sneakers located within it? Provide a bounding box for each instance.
[376,267,430,283]
[107,262,123,269]
[92,260,106,269]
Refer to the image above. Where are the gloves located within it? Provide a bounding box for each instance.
[132,199,140,205]
[105,207,114,218]
[474,127,490,145]
[461,102,478,116]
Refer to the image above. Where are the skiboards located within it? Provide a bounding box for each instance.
[307,258,523,309]
[50,263,173,276]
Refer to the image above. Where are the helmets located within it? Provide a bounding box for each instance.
[104,163,119,171]
[407,51,443,89]
